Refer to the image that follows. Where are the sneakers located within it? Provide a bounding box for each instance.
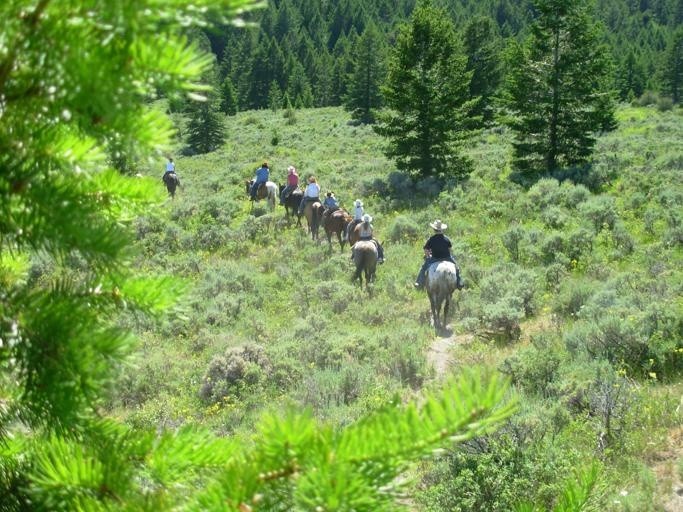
[378,259,385,263]
[458,282,464,290]
[413,282,423,290]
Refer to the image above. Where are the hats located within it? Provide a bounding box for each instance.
[430,219,448,231]
[361,214,372,222]
[354,199,363,207]
[262,162,268,166]
[325,190,334,197]
[309,176,315,182]
[286,166,295,172]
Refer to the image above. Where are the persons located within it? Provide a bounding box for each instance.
[321,190,341,226]
[350,212,376,260]
[412,218,465,293]
[295,175,325,219]
[248,163,269,202]
[161,158,176,179]
[276,165,300,207]
[344,199,365,241]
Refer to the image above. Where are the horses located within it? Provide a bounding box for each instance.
[319,205,349,254]
[422,253,458,330]
[344,214,364,247]
[279,181,304,226]
[164,172,179,199]
[300,189,324,240]
[244,177,280,212]
[350,238,385,292]
